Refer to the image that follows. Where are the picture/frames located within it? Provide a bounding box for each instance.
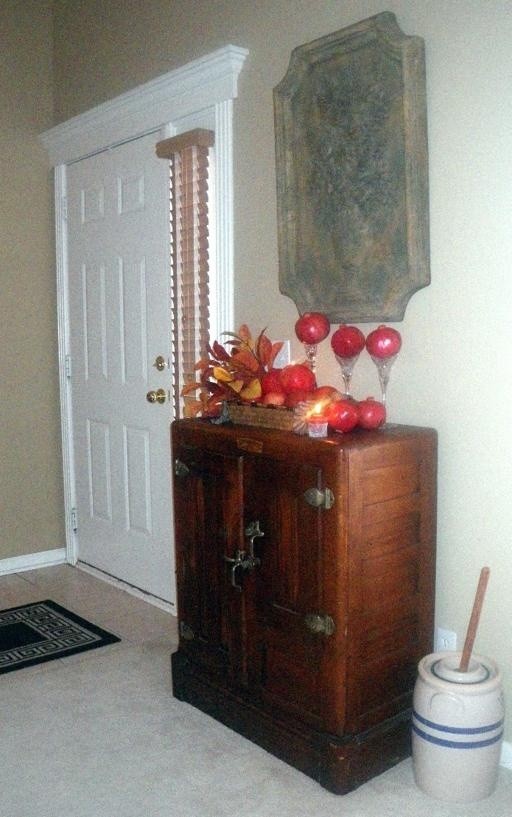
[272,11,432,325]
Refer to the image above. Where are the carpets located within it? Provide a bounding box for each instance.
[0,599,122,675]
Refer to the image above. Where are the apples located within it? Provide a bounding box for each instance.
[262,311,402,432]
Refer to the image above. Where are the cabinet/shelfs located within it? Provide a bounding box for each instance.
[166,418,438,797]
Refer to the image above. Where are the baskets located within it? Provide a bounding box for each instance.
[227,405,294,431]
